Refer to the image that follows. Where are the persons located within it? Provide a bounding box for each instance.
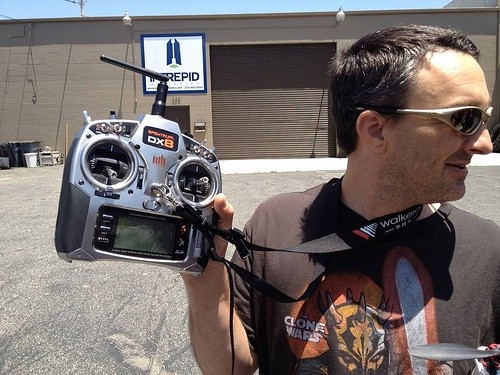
[179,23,500,375]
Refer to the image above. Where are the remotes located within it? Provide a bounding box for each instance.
[54,54,222,279]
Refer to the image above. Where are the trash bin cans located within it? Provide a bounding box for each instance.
[7,141,21,167]
[19,140,41,167]
[0,143,10,170]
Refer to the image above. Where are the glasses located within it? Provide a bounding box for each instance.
[357,105,494,137]
